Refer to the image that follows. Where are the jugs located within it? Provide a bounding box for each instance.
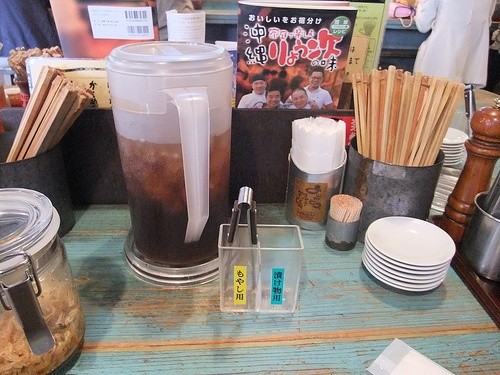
[106,44,233,286]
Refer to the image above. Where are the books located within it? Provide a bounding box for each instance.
[235,0,358,110]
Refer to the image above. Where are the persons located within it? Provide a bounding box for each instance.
[413,0,497,88]
[237,66,333,109]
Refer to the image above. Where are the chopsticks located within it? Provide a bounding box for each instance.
[352,66,467,167]
[6,43,94,161]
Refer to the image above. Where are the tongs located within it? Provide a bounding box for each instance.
[463,83,478,138]
[223,186,258,290]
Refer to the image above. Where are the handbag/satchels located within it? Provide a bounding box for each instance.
[388,2,416,28]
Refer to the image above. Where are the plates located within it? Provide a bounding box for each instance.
[441,128,467,167]
[360,216,456,291]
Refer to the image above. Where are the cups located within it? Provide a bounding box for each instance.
[1,128,77,233]
[13,77,30,106]
[464,189,500,282]
[164,9,205,43]
[342,135,446,244]
[325,213,360,251]
[284,151,346,230]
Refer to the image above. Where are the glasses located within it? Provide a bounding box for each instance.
[312,77,322,81]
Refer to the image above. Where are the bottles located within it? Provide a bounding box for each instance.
[0,187,85,374]
